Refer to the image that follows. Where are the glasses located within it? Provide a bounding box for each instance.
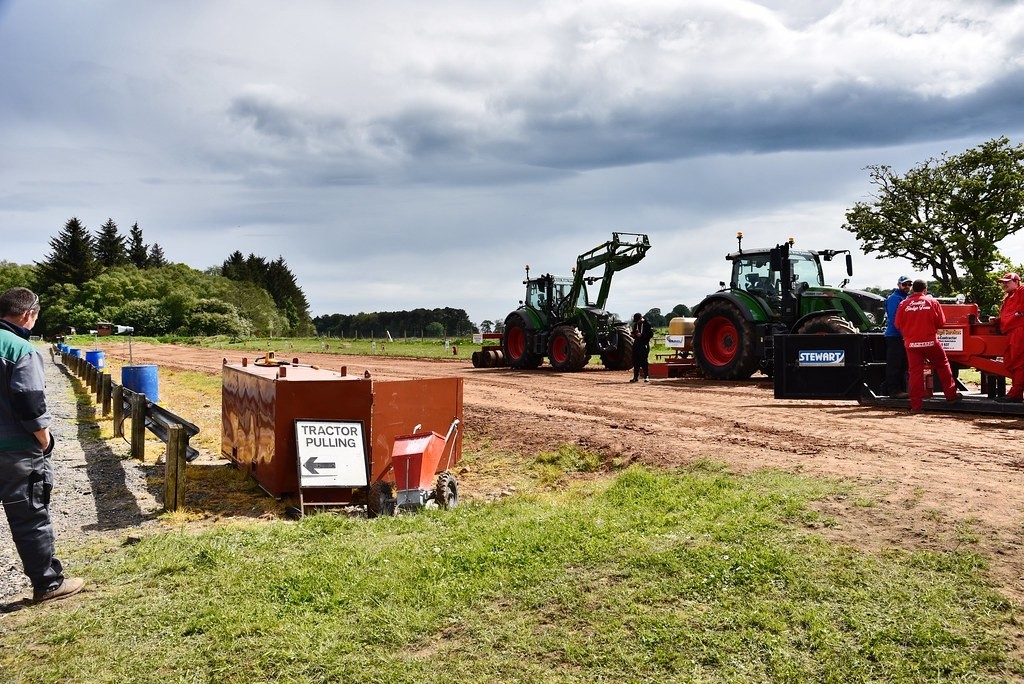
[27,293,39,312]
[902,283,912,287]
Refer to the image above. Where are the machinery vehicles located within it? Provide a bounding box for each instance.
[691,231,888,383]
[499,231,652,374]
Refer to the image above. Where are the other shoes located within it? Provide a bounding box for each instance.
[995,395,1023,402]
[644,378,649,382]
[911,409,923,413]
[946,393,962,404]
[891,391,908,398]
[630,379,637,382]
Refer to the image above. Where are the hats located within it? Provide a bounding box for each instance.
[996,273,1020,283]
[899,275,913,283]
[633,313,641,322]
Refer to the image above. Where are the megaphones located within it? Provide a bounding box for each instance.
[115,325,128,334]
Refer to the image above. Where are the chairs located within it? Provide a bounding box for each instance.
[744,273,775,296]
[539,294,547,306]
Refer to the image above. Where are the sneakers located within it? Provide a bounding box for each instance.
[34,578,85,604]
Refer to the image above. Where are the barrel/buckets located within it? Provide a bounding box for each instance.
[63,346,70,353]
[86,350,106,373]
[57,344,64,349]
[121,365,160,417]
[70,349,82,359]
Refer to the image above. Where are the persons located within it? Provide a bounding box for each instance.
[894,279,964,412]
[0,287,85,604]
[989,273,1024,404]
[630,313,654,383]
[884,276,913,398]
